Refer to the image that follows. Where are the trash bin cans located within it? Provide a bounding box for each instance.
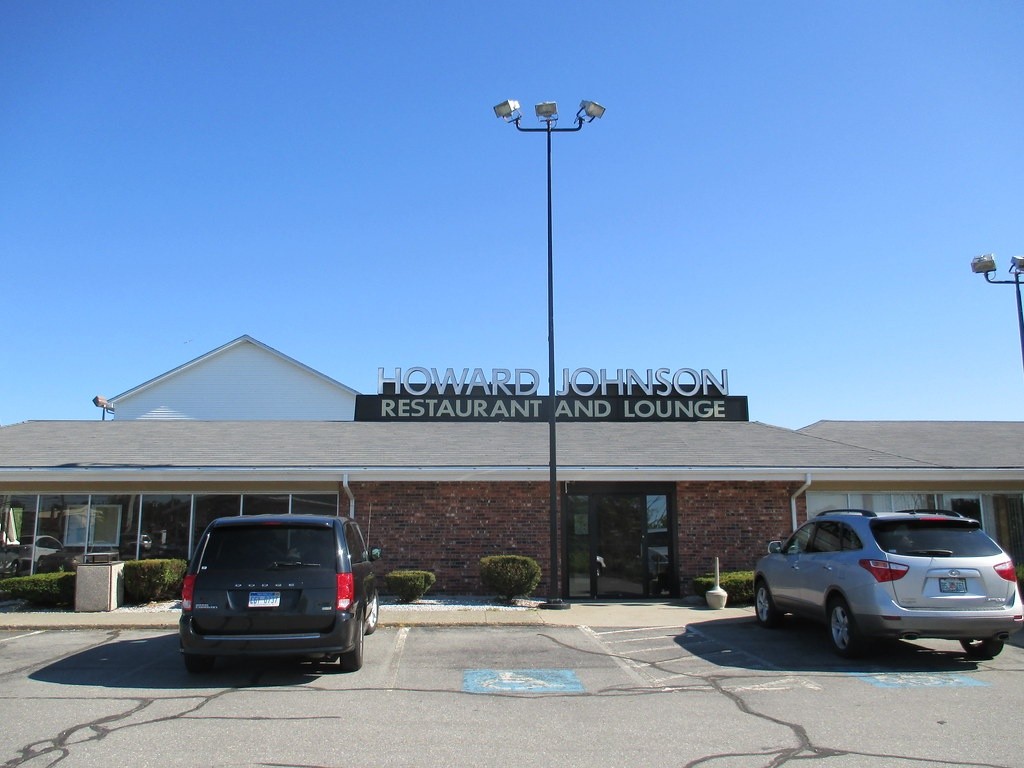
[75,552,126,612]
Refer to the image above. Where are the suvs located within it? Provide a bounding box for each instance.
[177,512,384,673]
[749,508,1024,660]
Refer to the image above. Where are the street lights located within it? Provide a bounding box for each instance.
[93,396,114,422]
[971,255,1024,374]
[491,96,606,613]
